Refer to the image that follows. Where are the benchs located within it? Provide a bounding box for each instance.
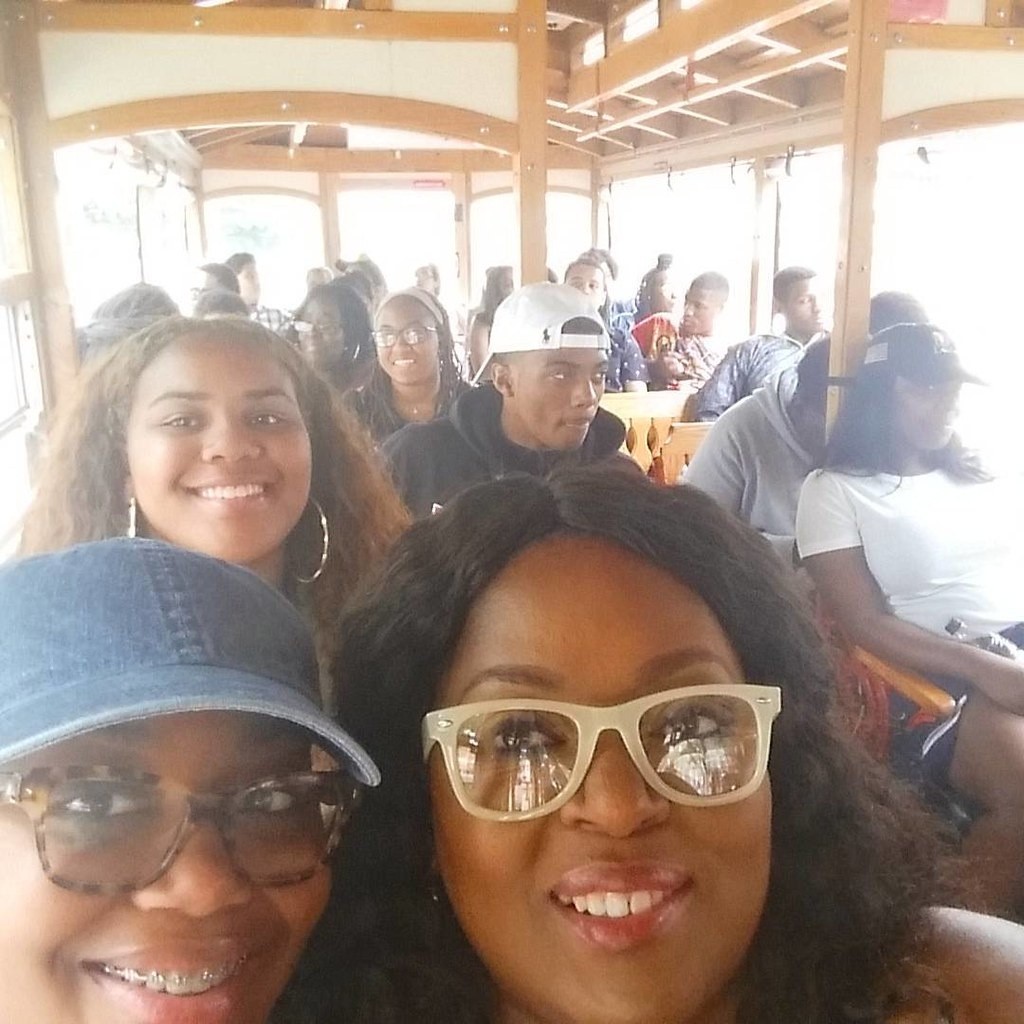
[595,389,715,485]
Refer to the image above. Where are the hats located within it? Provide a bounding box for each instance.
[470,281,610,384]
[0,534,383,788]
[861,322,984,386]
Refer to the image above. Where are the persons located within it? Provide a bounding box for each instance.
[0,241,1021,1024]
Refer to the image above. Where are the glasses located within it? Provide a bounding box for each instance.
[370,325,437,347]
[0,766,363,894]
[293,318,341,336]
[419,683,784,824]
